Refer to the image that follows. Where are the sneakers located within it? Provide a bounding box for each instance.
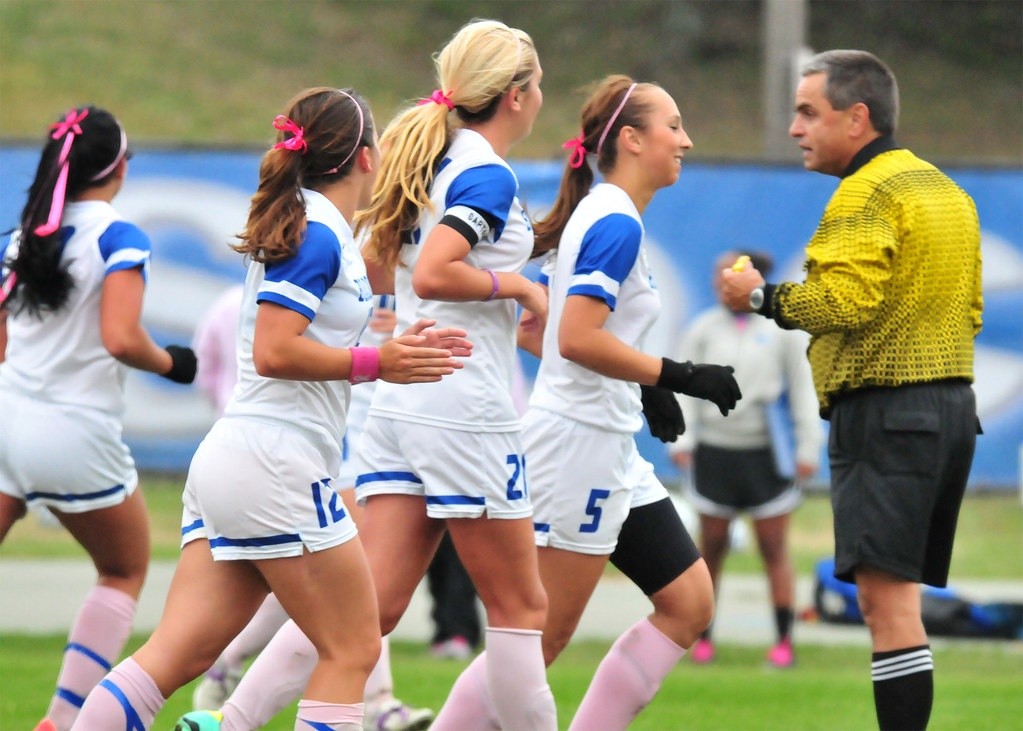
[361,695,435,731]
[192,666,244,711]
[174,709,224,731]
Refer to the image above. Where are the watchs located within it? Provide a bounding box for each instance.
[749,283,765,312]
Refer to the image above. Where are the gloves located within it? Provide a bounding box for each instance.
[658,357,743,416]
[159,346,198,384]
[640,383,685,444]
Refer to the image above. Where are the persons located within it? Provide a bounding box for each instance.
[721,51,985,731]
[676,251,825,665]
[428,531,482,661]
[193,214,435,731]
[58,86,382,731]
[1,105,198,731]
[426,77,744,731]
[177,17,558,731]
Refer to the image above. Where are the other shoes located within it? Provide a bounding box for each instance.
[767,638,793,667]
[430,635,471,660]
[692,639,713,664]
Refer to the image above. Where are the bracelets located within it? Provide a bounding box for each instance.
[349,346,378,385]
[484,269,498,301]
